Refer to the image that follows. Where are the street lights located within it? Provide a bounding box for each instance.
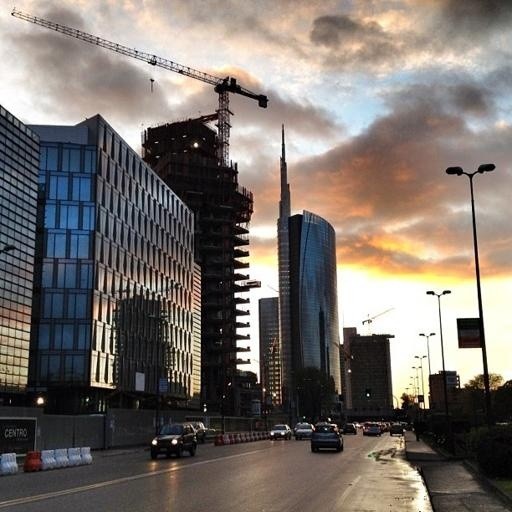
[425,290,451,417]
[444,160,495,430]
[404,355,427,410]
[418,333,436,375]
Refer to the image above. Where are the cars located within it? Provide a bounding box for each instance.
[270,420,415,453]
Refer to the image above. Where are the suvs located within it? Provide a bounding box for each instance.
[188,421,207,443]
[150,423,198,458]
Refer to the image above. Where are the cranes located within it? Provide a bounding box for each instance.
[9,10,271,168]
[361,308,394,335]
